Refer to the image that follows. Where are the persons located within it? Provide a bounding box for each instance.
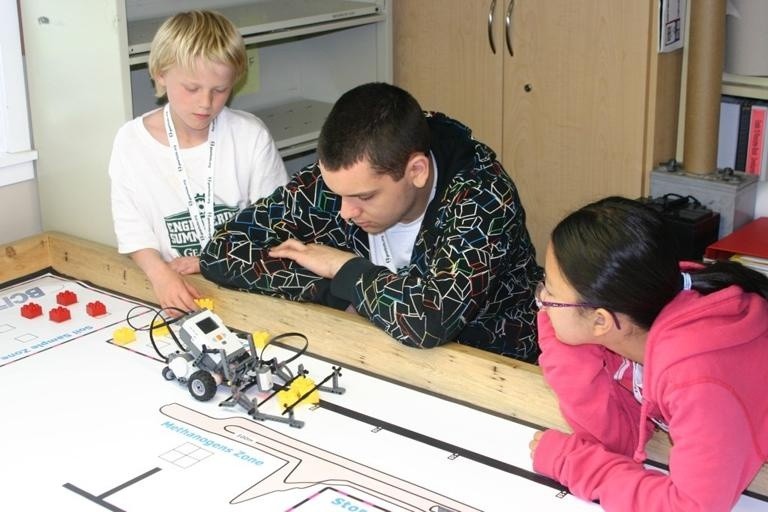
[195,80,550,366]
[526,189,766,510]
[109,8,291,320]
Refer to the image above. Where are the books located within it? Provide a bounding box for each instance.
[709,66,767,183]
[696,214,767,279]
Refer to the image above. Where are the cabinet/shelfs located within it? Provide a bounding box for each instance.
[391,0,689,269]
[17,1,395,252]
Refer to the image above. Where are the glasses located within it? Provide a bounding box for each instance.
[535,281,622,330]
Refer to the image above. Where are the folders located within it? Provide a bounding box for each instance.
[703,216,768,280]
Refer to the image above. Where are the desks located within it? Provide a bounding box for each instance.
[650,164,759,239]
[1,229,768,512]
